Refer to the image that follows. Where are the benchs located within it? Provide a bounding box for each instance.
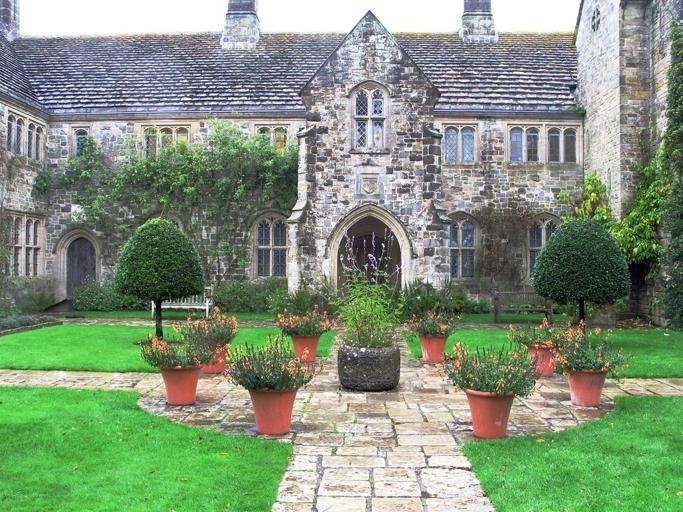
[493,289,554,327]
[150,283,215,318]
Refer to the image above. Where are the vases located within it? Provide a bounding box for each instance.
[339,344,400,390]
[419,334,446,364]
[292,335,318,362]
[160,365,202,404]
[566,368,607,405]
[201,345,226,374]
[250,388,297,434]
[529,344,554,377]
[465,388,514,438]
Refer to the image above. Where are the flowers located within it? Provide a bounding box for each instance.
[225,337,323,389]
[312,227,423,348]
[440,341,542,399]
[508,318,572,348]
[551,318,634,383]
[141,334,214,368]
[278,305,338,337]
[172,308,237,363]
[402,306,462,336]
